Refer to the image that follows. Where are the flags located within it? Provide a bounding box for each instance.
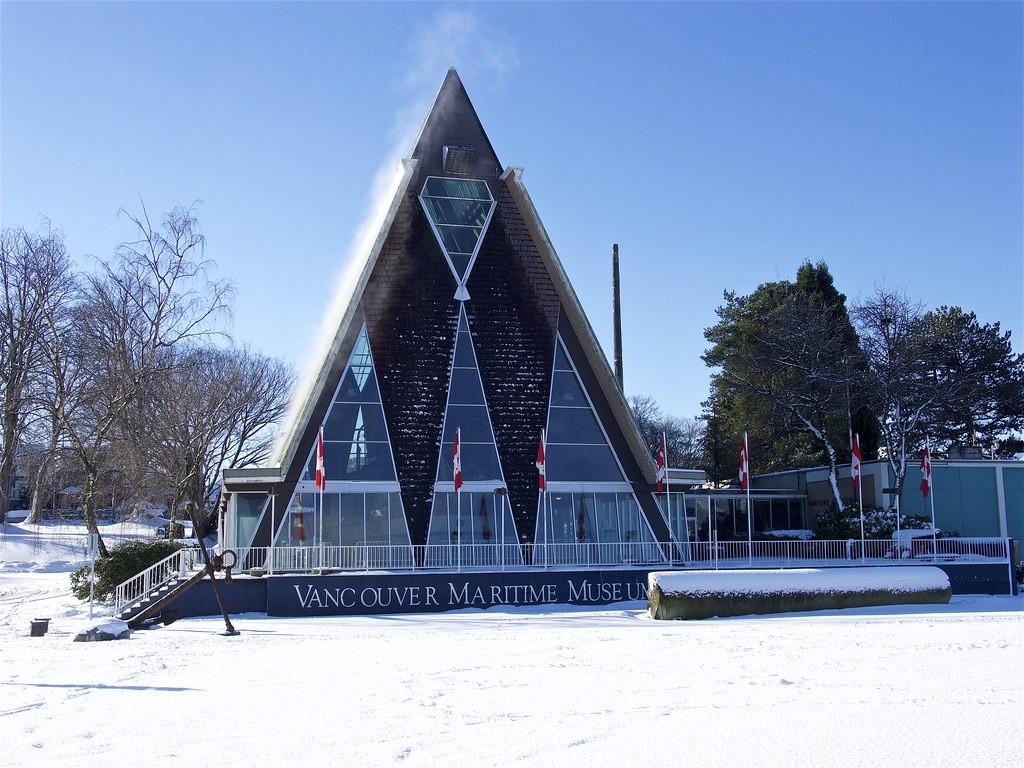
[577,499,586,543]
[315,430,326,491]
[452,431,463,492]
[293,495,303,538]
[738,438,747,489]
[919,444,931,498]
[479,495,491,540]
[536,434,545,494]
[657,440,664,493]
[850,436,862,489]
